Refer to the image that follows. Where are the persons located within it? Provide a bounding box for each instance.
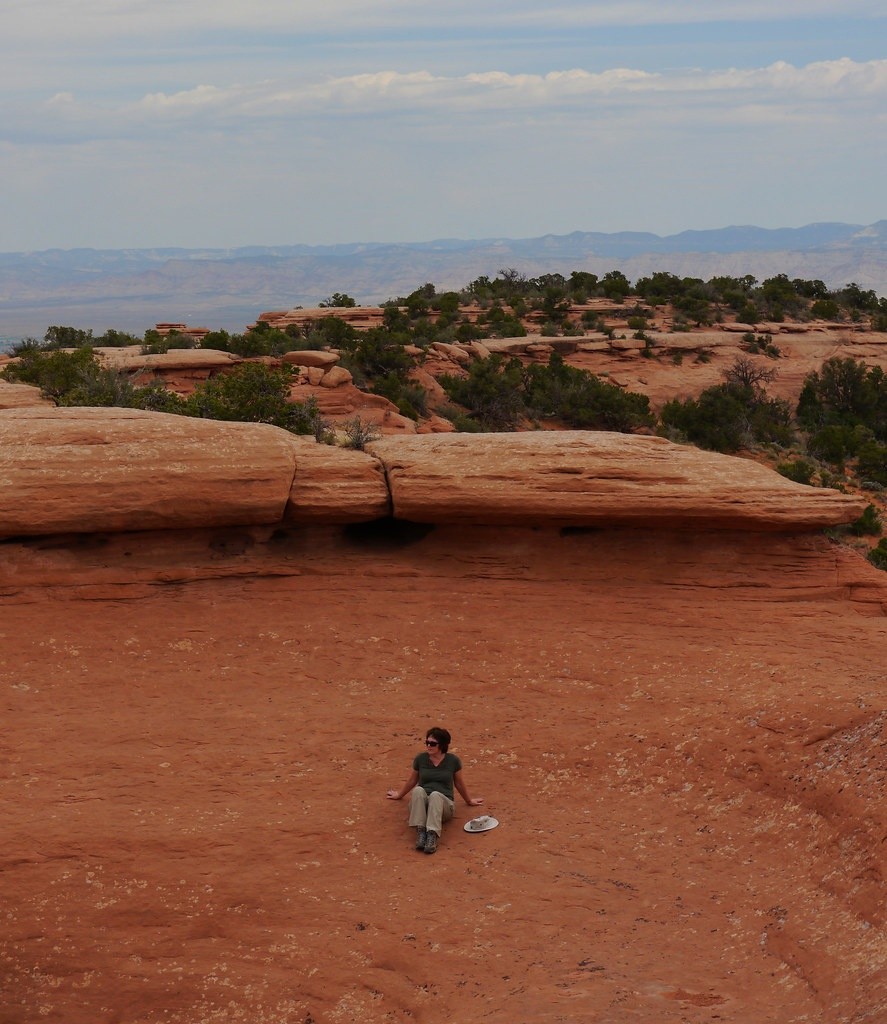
[387,728,485,852]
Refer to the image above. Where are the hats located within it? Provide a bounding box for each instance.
[464,816,498,832]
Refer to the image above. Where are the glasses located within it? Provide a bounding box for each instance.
[426,740,439,747]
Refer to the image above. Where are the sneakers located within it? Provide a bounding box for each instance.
[424,831,437,853]
[416,828,426,848]
